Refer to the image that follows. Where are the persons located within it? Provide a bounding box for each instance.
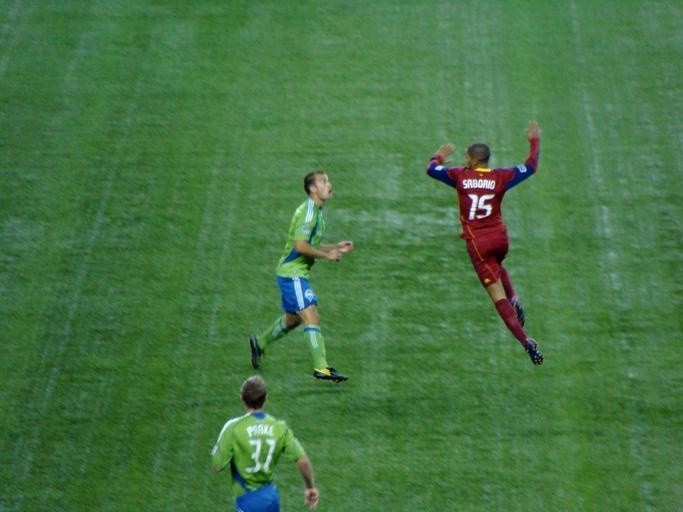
[248,171,352,380]
[209,375,319,512]
[425,119,545,366]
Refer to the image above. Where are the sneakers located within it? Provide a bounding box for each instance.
[510,296,524,328]
[249,333,262,369]
[525,338,543,366]
[313,366,347,383]
